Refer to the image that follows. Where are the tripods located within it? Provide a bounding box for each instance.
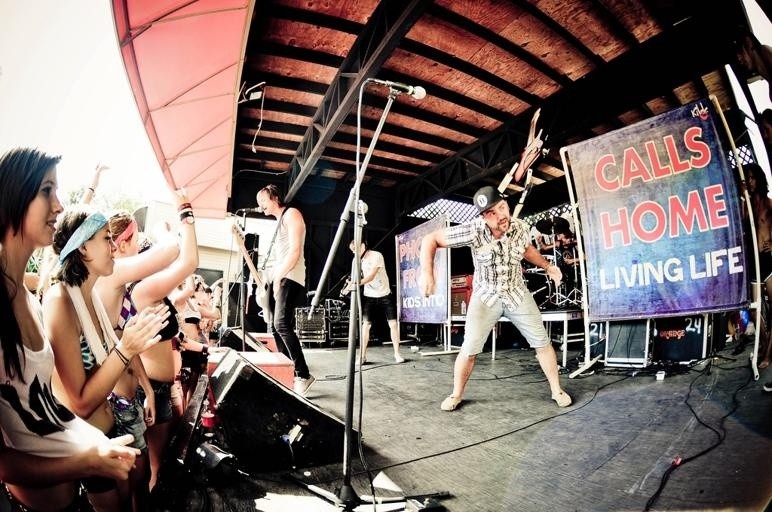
[282,202,450,512]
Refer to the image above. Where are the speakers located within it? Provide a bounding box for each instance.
[217,326,272,352]
[205,348,363,481]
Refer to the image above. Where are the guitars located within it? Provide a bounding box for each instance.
[231,222,273,324]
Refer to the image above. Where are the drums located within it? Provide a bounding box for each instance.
[523,271,556,310]
[534,254,555,272]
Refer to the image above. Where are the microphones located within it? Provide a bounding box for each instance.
[239,206,265,211]
[375,79,428,99]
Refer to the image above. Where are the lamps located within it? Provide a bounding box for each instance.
[245,84,262,101]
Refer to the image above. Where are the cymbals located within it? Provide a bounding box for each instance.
[562,241,577,246]
[535,216,570,234]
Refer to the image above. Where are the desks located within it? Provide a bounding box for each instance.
[443,310,584,367]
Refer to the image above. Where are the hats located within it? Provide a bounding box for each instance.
[473,186,504,217]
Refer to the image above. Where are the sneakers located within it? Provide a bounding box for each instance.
[355,357,366,365]
[552,390,572,407]
[394,355,405,363]
[294,374,315,395]
[441,394,461,410]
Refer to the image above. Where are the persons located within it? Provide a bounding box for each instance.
[256,183,317,397]
[421,186,572,412]
[349,238,404,366]
[739,163,772,369]
[0,147,222,512]
[538,229,586,274]
